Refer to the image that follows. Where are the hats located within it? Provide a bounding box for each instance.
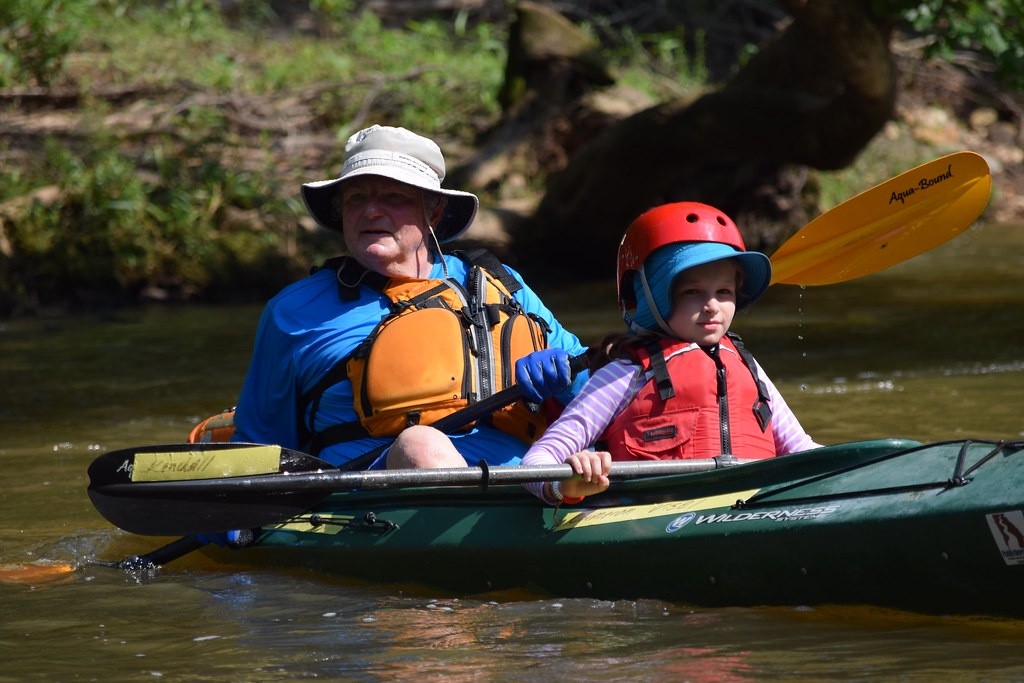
[631,241,772,329]
[301,124,478,248]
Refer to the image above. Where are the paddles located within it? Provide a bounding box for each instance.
[85,443,762,534]
[110,150,991,571]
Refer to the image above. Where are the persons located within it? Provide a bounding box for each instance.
[521,202,827,505]
[188,124,589,551]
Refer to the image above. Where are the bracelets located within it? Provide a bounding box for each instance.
[552,481,585,504]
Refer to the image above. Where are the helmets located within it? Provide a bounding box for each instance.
[617,202,746,311]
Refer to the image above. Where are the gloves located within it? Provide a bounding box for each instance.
[515,348,572,404]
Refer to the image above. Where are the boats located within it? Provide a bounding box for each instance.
[189,416,1024,627]
